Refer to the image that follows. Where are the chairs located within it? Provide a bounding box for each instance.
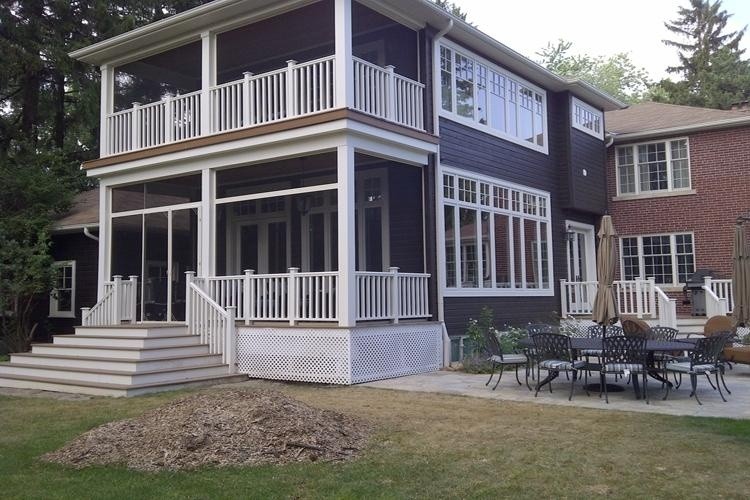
[483,317,737,405]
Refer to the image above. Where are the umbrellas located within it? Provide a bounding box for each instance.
[731,216,750,327]
[592,209,620,339]
